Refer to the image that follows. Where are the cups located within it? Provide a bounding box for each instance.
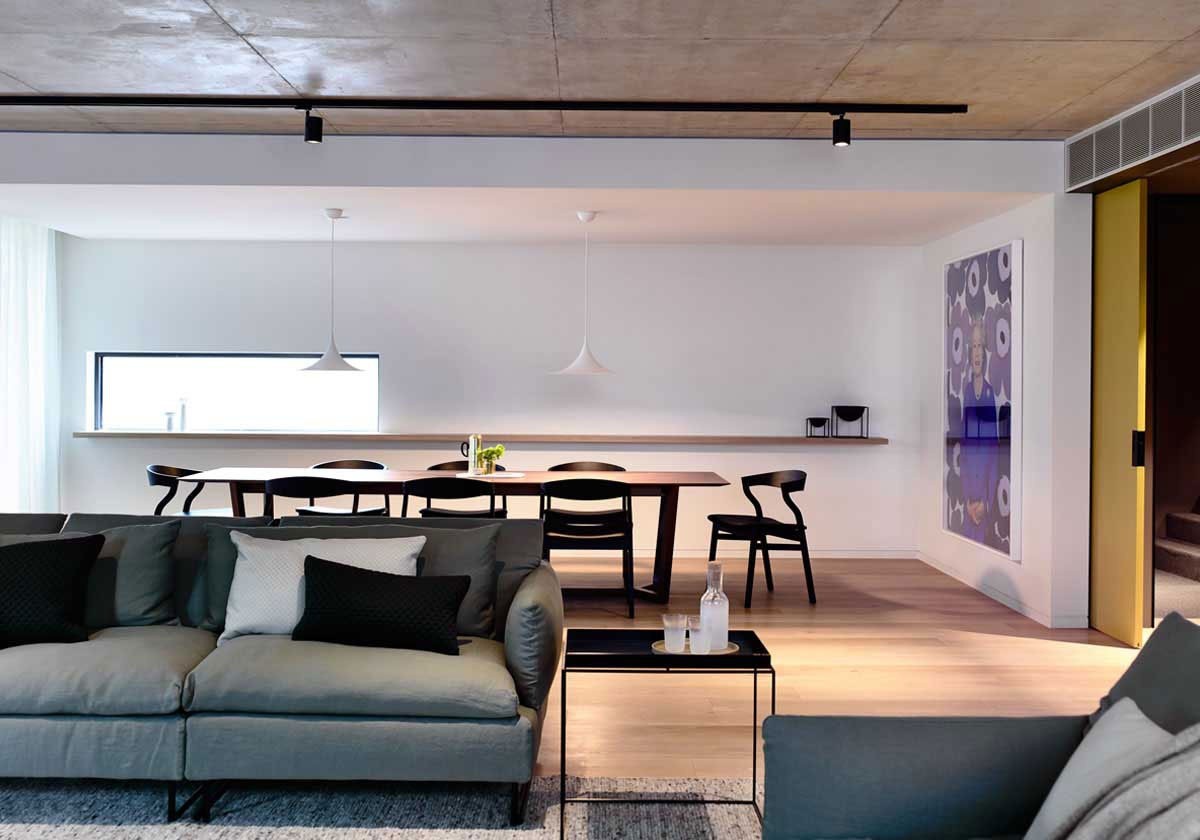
[687,615,710,654]
[663,613,687,654]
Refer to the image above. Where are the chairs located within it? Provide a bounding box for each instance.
[397,477,496,518]
[424,458,510,519]
[540,461,635,599]
[145,464,206,514]
[706,468,817,608]
[264,475,374,518]
[294,458,391,516]
[538,478,636,619]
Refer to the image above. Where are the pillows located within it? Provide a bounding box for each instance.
[198,519,503,642]
[0,533,105,650]
[0,516,182,628]
[290,554,472,657]
[215,529,427,648]
[1022,696,1175,840]
[1081,610,1199,749]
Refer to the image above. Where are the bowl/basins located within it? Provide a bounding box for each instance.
[472,468,489,475]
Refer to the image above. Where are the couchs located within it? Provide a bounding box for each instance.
[0,512,566,824]
[761,714,1100,839]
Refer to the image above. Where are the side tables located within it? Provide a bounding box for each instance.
[559,626,777,840]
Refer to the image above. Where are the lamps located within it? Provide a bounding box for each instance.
[295,104,323,145]
[299,208,365,371]
[828,111,852,147]
[545,211,618,376]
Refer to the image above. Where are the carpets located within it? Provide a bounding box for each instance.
[1,772,776,838]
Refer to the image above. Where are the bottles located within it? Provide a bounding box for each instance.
[699,560,730,650]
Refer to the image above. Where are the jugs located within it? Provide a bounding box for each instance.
[461,433,482,474]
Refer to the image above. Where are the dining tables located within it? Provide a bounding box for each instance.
[177,467,732,605]
